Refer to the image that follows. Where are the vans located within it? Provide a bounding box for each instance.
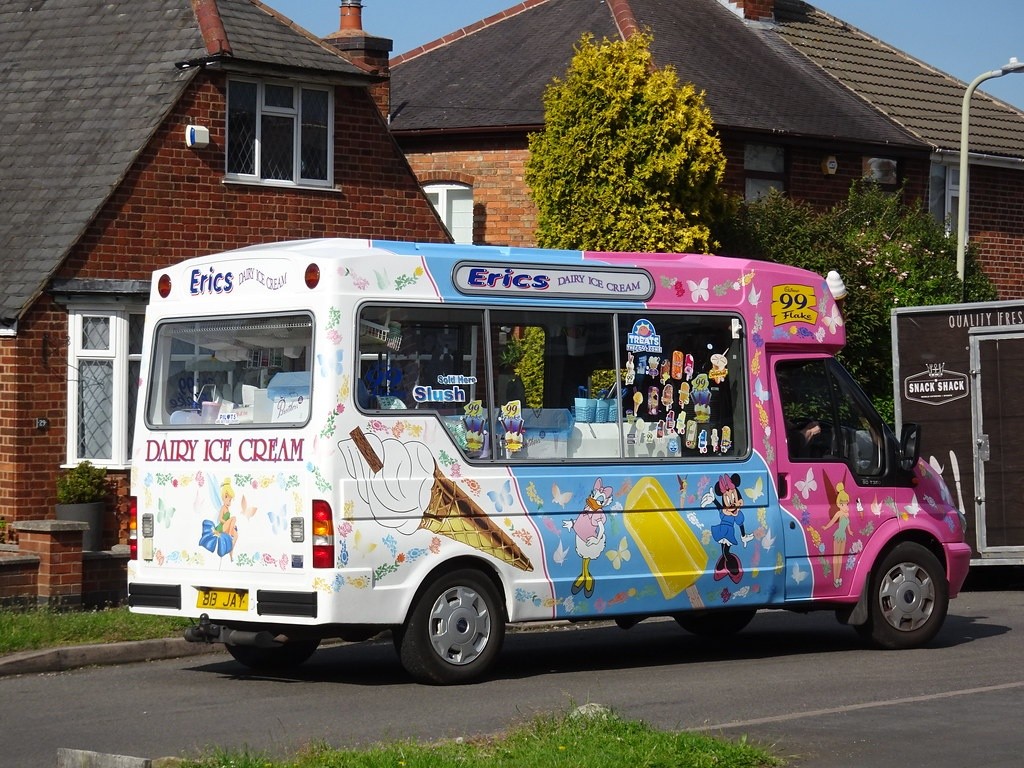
[124,237,964,687]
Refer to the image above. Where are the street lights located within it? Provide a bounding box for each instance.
[953,60,1024,301]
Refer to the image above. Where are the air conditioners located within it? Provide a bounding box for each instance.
[862,157,898,184]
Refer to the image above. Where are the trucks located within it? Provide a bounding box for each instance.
[886,302,1024,566]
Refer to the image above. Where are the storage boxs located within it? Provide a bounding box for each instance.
[241,370,311,422]
[446,406,683,460]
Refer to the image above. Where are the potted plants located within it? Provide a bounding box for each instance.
[55,460,118,550]
